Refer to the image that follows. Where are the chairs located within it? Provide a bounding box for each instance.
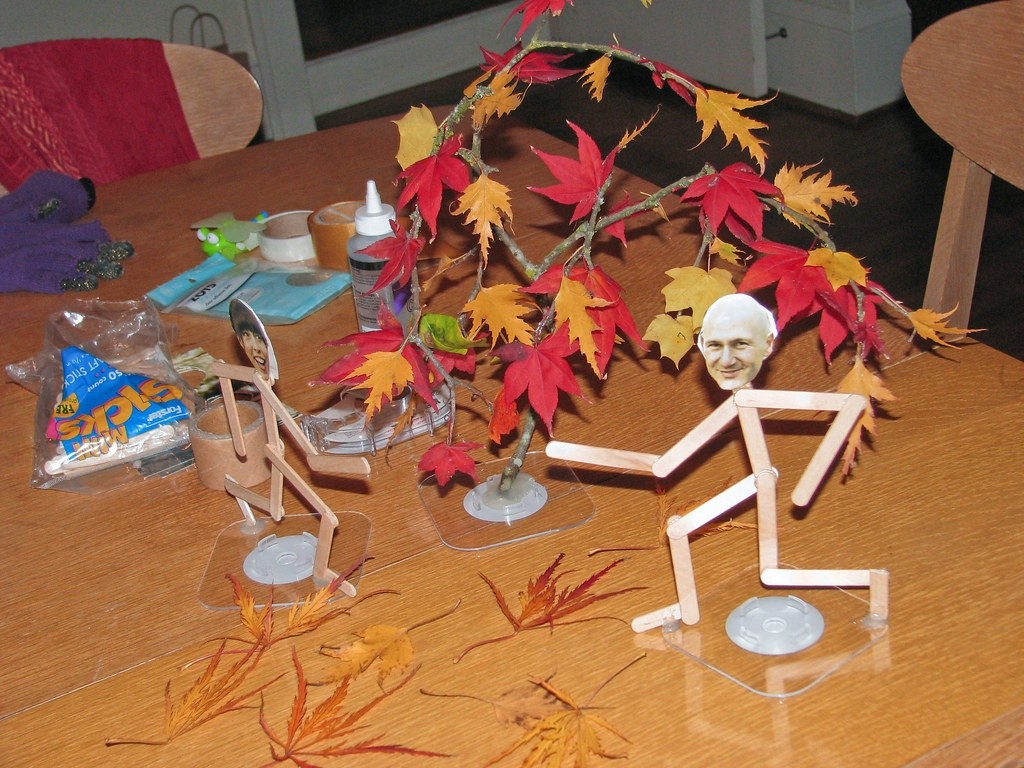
[900,1,1024,338]
[0,38,264,194]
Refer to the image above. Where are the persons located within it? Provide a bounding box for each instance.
[699,293,774,391]
[229,299,270,381]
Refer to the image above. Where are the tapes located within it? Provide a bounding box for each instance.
[256,210,316,263]
[306,200,368,272]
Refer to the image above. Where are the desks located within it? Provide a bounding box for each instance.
[0,104,1024,768]
